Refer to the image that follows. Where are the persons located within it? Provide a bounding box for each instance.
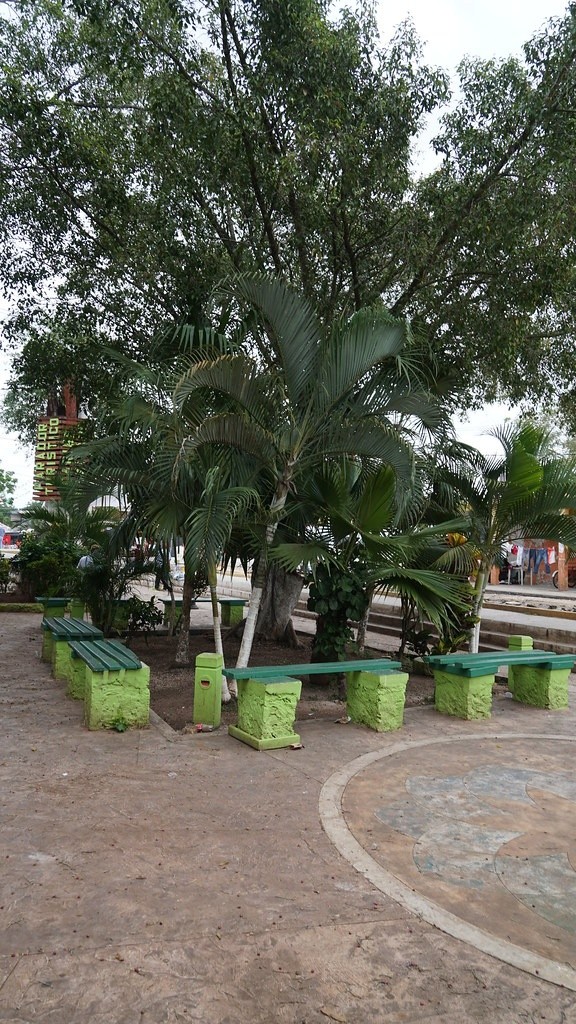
[76,545,99,567]
[154,544,173,591]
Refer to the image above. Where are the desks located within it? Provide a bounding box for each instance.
[508,566,534,585]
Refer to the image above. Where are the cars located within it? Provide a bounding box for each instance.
[0,522,26,547]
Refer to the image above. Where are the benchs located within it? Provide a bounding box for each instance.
[2,545,13,548]
[421,648,576,721]
[64,638,152,729]
[221,656,410,751]
[159,597,250,630]
[40,615,104,678]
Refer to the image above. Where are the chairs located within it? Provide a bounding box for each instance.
[35,596,131,630]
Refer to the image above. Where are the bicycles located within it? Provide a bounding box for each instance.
[551,555,575,589]
